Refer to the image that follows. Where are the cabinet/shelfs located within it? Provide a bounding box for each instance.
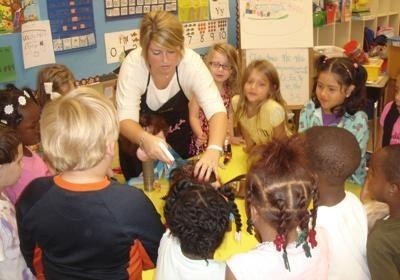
[313,1,400,54]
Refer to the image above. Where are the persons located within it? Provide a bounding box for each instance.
[0,86,56,206]
[185,42,246,158]
[155,178,238,279]
[14,86,165,280]
[116,8,229,183]
[230,58,294,157]
[224,141,319,279]
[0,123,36,280]
[38,64,77,106]
[292,125,370,280]
[365,144,400,279]
[359,68,400,204]
[297,54,371,188]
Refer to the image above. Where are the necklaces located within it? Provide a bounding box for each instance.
[157,72,171,80]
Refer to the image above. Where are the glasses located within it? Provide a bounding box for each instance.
[209,61,234,70]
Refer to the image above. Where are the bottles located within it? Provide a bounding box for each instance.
[344,40,369,65]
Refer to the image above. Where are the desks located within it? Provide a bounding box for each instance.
[366,74,389,117]
[126,137,375,280]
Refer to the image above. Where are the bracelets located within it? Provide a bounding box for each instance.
[206,144,223,152]
[139,132,149,152]
